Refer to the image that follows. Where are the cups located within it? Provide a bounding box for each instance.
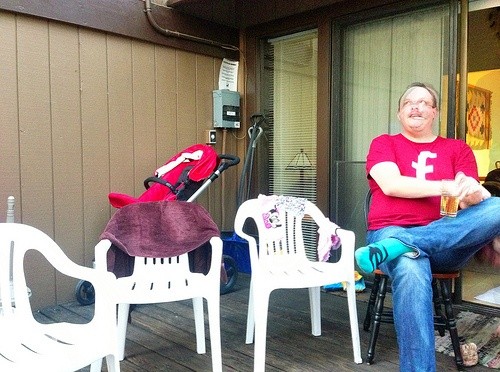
[439,180,459,217]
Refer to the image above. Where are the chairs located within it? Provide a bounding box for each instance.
[234,196,363,372]
[90,202,223,372]
[0,221,120,372]
[364,190,465,372]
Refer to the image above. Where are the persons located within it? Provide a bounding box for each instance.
[355,82,500,372]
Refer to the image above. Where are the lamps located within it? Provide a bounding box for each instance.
[285,148,317,198]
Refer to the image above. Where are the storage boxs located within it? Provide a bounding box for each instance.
[221,234,259,273]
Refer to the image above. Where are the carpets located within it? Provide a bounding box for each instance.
[431,311,500,368]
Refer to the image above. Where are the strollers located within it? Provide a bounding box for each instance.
[73,141,240,306]
